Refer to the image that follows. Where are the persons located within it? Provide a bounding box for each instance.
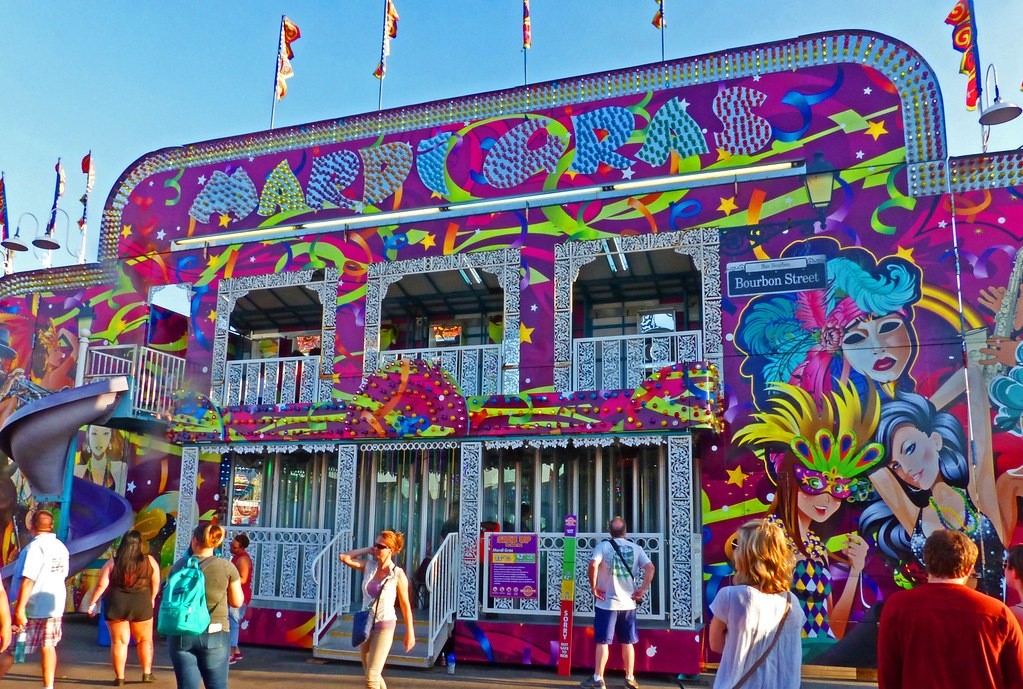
[88,530,161,686]
[0,510,69,689]
[709,519,806,689]
[580,517,655,689]
[182,536,222,558]
[166,524,245,689]
[340,531,415,689]
[416,540,431,582]
[1005,544,1023,633]
[878,528,1023,689]
[520,502,532,532]
[440,500,460,539]
[0,572,13,653]
[227,534,253,664]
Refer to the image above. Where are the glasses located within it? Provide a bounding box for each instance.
[230,542,242,550]
[374,542,391,550]
[731,538,741,550]
[1004,559,1008,568]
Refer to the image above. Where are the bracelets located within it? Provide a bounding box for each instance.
[89,602,95,606]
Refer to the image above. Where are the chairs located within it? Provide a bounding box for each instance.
[409,557,430,610]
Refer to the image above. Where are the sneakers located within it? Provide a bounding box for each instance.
[579,673,607,689]
[624,678,638,689]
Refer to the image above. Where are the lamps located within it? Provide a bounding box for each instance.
[0,213,44,268]
[174,159,806,246]
[980,64,1023,153]
[32,208,86,263]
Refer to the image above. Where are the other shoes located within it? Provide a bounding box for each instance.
[113,678,125,686]
[229,655,237,665]
[142,672,159,682]
[234,653,243,660]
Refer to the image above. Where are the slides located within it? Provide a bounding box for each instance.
[0,375,136,603]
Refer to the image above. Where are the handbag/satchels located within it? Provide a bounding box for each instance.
[352,609,375,647]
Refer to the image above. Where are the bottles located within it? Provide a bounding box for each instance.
[13,628,27,664]
[447,653,455,674]
[441,652,446,666]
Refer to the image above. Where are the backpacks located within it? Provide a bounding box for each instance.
[157,556,226,636]
